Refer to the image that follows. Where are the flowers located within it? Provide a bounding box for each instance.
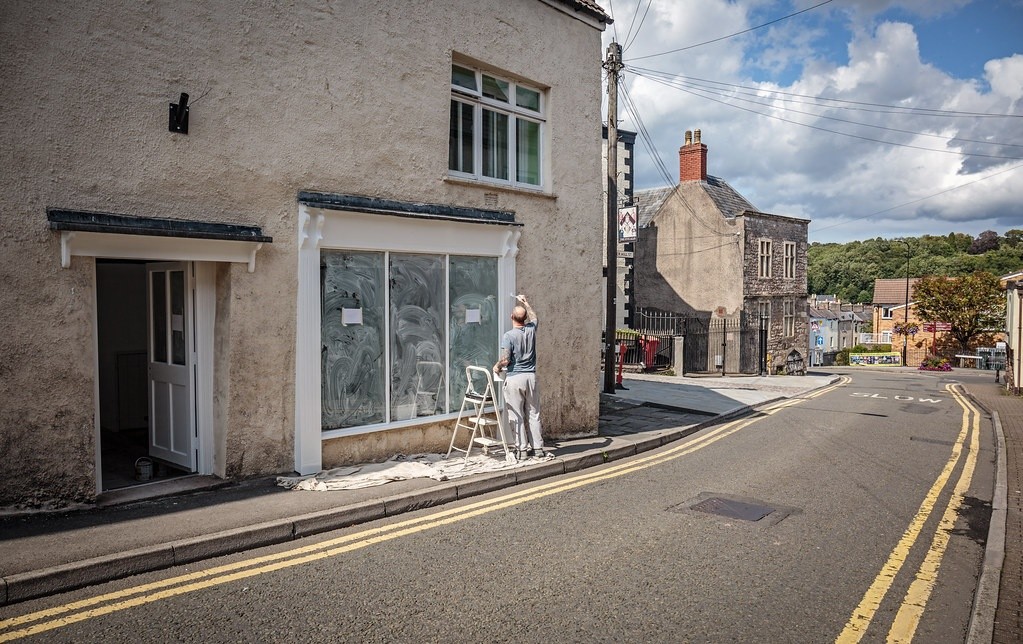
[893,322,906,333]
[906,323,919,334]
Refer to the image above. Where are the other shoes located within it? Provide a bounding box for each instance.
[515,450,527,460]
[528,449,544,457]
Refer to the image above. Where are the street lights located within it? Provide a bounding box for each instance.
[893,240,909,366]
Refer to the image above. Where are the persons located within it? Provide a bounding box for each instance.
[493,294,545,460]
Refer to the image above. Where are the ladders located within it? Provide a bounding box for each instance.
[445,365,509,467]
[411,361,456,419]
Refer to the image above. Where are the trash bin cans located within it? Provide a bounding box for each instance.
[639,334,660,367]
